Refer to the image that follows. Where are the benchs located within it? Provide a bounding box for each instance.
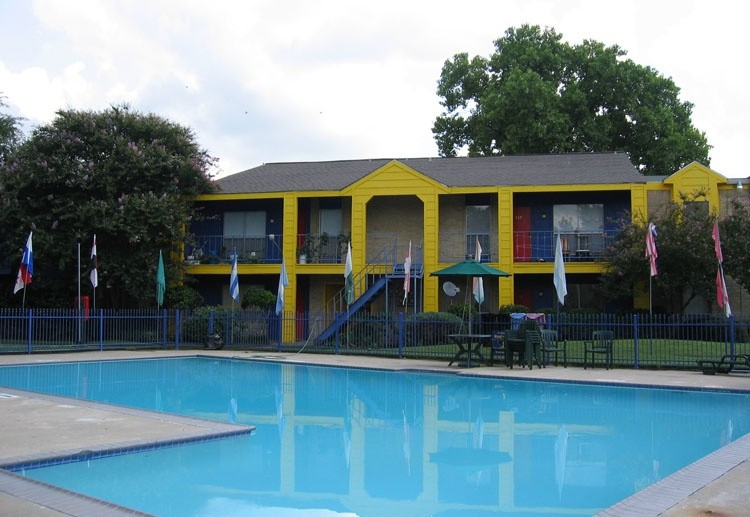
[697,353,750,374]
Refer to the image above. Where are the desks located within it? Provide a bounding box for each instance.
[446,334,493,367]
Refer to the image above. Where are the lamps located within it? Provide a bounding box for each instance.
[541,211,548,220]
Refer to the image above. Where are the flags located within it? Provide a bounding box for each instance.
[711,219,731,318]
[473,236,484,304]
[230,250,240,300]
[156,251,165,304]
[554,231,567,306]
[404,244,411,293]
[87,236,99,288]
[14,231,33,294]
[345,242,354,305]
[276,262,288,315]
[645,223,659,276]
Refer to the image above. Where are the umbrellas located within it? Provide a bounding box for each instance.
[431,259,513,335]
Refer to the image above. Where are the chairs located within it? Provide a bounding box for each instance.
[584,330,614,370]
[560,237,572,261]
[577,235,590,261]
[489,320,567,370]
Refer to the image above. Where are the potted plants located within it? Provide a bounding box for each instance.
[298,232,353,263]
